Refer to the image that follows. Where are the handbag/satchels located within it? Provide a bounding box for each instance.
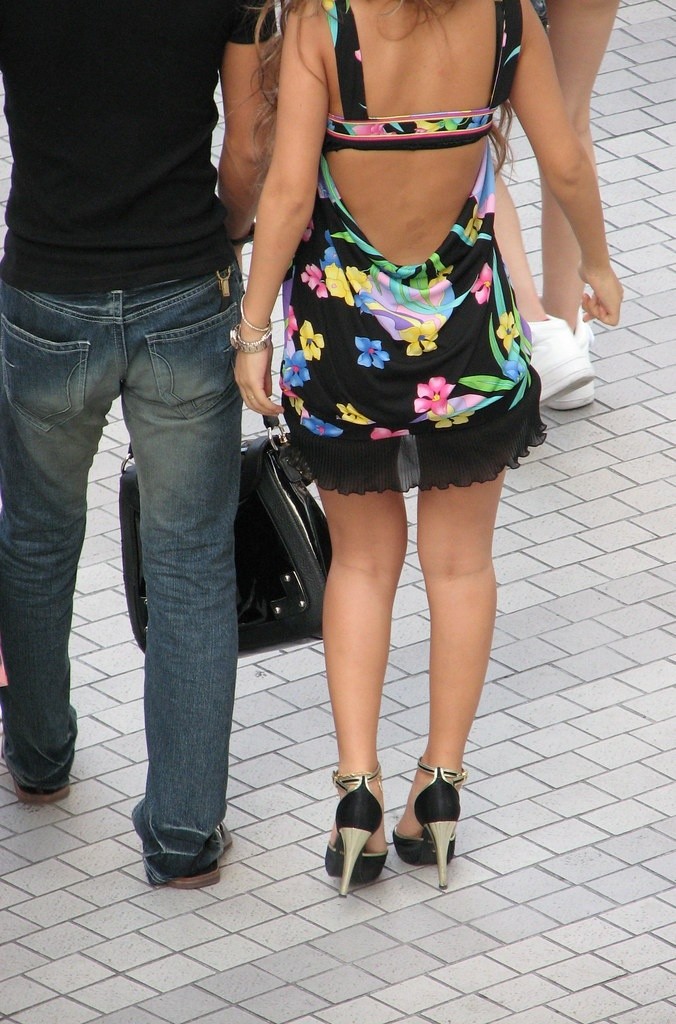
[119,432,331,660]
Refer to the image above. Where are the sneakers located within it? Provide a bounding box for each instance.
[539,313,594,410]
[520,314,593,407]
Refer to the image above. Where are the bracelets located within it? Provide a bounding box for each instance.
[240,294,270,331]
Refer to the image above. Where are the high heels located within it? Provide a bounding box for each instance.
[326,767,388,898]
[392,756,466,889]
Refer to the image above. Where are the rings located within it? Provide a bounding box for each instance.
[248,396,254,399]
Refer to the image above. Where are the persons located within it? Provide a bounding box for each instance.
[234,0,624,895]
[0,0,281,889]
[492,0,620,410]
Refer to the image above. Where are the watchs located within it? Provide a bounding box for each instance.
[230,224,255,245]
[230,325,272,353]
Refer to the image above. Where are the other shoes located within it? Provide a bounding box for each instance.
[2,734,70,804]
[166,817,232,888]
